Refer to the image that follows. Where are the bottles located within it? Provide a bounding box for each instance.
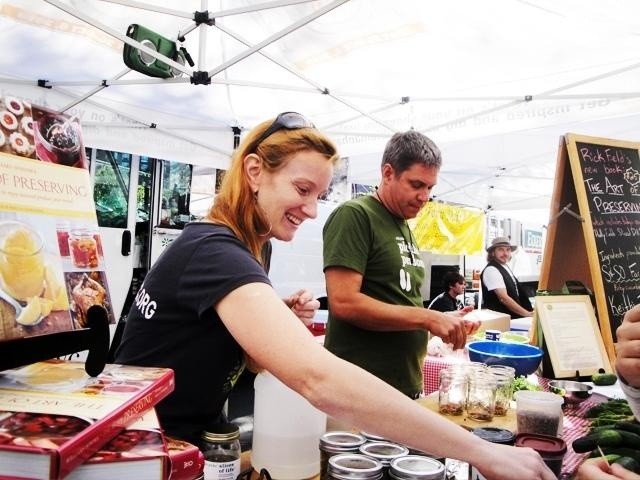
[468,390,568,479]
[34,112,80,166]
[438,363,515,423]
[319,432,446,480]
[54,221,103,268]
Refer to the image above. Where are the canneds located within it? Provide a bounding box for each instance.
[438,361,516,422]
[484,330,500,341]
[319,431,445,480]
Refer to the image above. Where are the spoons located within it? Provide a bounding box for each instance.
[1,287,47,327]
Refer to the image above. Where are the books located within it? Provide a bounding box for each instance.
[0,359,176,480]
[65,404,173,480]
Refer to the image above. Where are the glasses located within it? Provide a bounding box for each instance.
[248,111,315,153]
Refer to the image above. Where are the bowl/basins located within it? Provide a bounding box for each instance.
[465,332,545,379]
[547,380,593,406]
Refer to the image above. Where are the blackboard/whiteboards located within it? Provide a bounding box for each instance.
[564,133,640,374]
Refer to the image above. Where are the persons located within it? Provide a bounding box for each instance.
[572,303,640,480]
[320,126,470,399]
[480,235,535,320]
[425,270,466,311]
[108,111,563,480]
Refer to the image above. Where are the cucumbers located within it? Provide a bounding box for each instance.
[571,400,639,474]
[591,374,617,386]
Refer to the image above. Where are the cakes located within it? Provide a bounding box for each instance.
[0,97,34,155]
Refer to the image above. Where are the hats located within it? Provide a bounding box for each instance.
[487,237,518,252]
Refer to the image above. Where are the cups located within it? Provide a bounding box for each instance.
[0,221,46,300]
